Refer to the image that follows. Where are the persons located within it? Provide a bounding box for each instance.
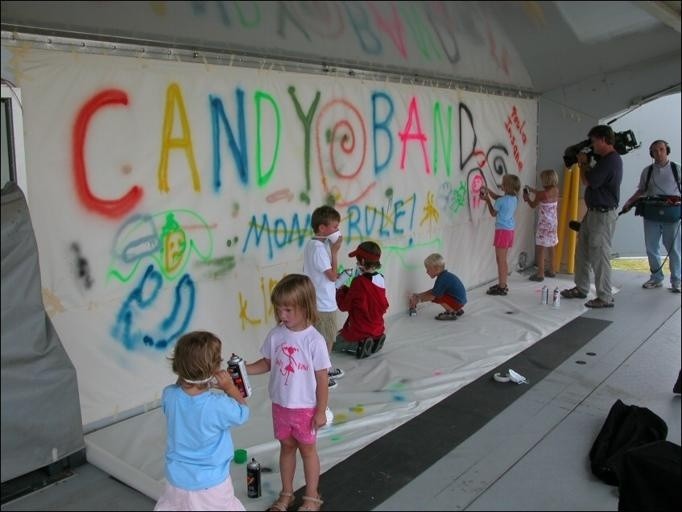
[303,206,354,390]
[335,241,390,360]
[226,273,333,512]
[479,174,521,295]
[409,254,468,321]
[623,140,681,294]
[559,126,623,308]
[152,331,251,512]
[523,169,559,282]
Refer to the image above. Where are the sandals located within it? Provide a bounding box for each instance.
[529,274,545,283]
[456,310,465,316]
[486,284,510,297]
[436,311,456,322]
[298,495,324,512]
[269,489,298,511]
[545,270,555,277]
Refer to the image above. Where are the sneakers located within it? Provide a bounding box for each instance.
[358,336,374,359]
[375,334,386,352]
[584,298,616,310]
[328,367,344,378]
[670,282,681,294]
[562,287,587,299]
[329,380,338,388]
[642,278,665,289]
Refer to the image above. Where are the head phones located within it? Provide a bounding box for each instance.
[650,140,671,158]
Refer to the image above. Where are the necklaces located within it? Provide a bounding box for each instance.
[182,375,213,385]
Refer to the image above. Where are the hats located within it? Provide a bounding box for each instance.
[348,245,382,260]
